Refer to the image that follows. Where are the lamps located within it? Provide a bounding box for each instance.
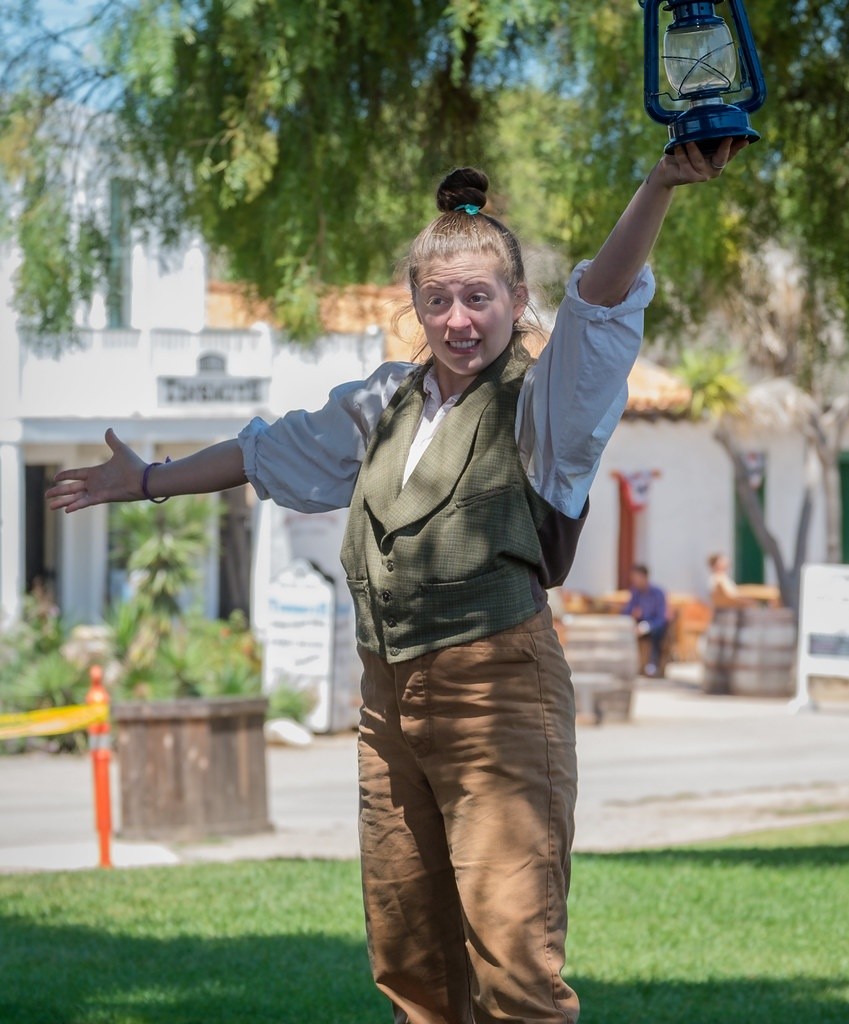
[634,0,775,159]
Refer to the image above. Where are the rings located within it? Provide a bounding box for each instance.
[711,162,726,170]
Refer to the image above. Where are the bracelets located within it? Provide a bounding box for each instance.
[142,457,173,504]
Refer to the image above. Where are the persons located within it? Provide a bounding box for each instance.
[621,565,667,677]
[706,552,747,610]
[42,135,752,1024]
